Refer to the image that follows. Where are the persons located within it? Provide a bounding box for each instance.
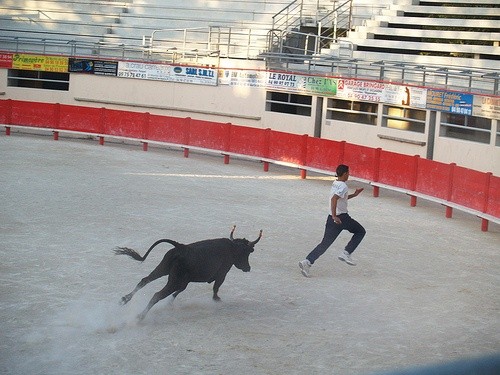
[298,164,367,279]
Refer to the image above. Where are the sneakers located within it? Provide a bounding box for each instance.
[299,261,312,278]
[338,252,357,266]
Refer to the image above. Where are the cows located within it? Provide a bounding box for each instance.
[112,225,263,324]
